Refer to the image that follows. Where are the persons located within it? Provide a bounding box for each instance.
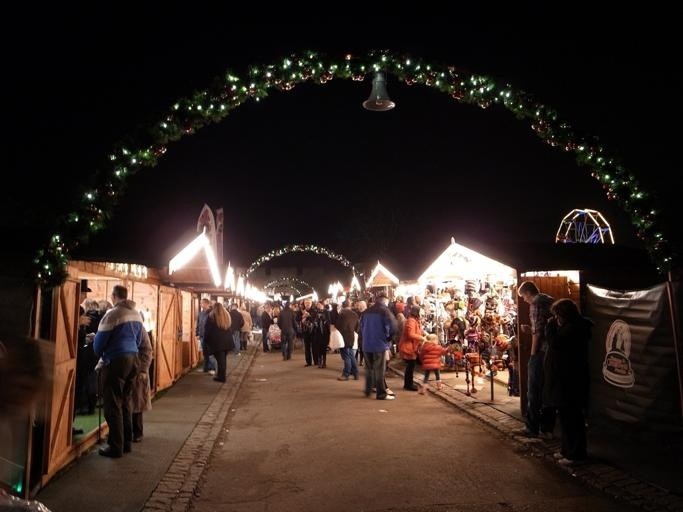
[94,299,113,408]
[511,279,557,437]
[90,282,142,460]
[397,305,428,392]
[74,299,101,415]
[388,293,421,358]
[69,277,93,437]
[417,334,451,395]
[256,292,369,381]
[541,298,594,468]
[194,297,253,384]
[358,293,396,401]
[125,324,154,443]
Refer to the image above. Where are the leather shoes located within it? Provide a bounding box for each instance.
[213,376,224,382]
[405,385,418,391]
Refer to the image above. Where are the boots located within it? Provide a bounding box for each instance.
[418,384,428,395]
[436,380,443,391]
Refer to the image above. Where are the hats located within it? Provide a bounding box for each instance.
[81,280,91,292]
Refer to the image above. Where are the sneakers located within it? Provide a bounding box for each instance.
[363,387,396,400]
[511,425,536,436]
[337,375,348,381]
[553,452,563,459]
[99,446,114,457]
[72,427,83,434]
[558,457,585,464]
